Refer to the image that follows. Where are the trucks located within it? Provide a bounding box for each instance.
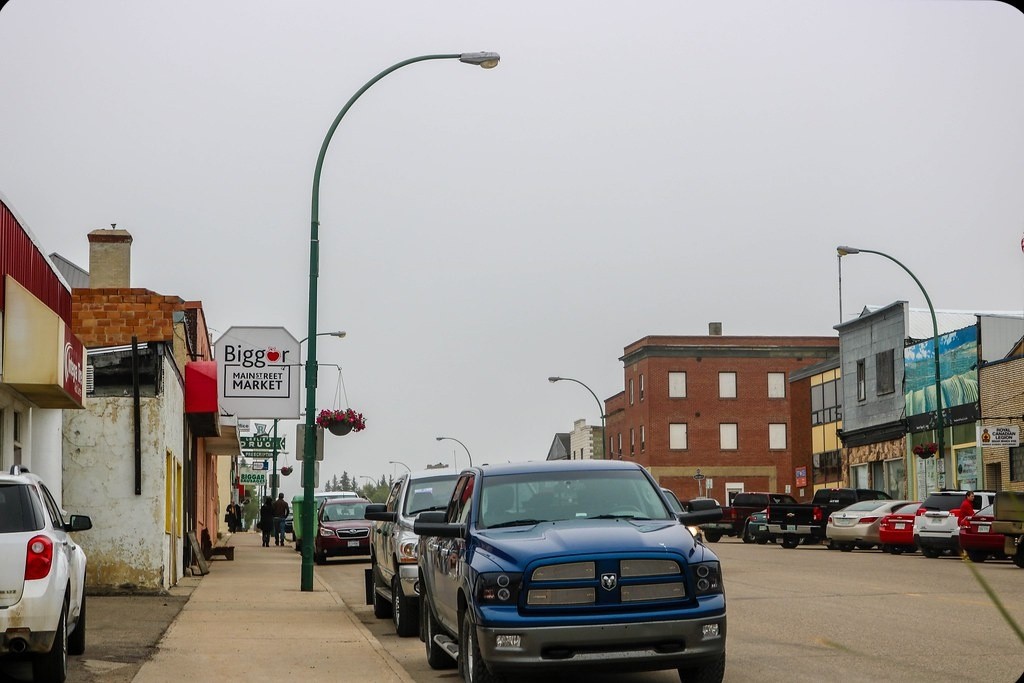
[991,490,1024,569]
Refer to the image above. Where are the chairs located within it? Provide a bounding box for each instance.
[410,491,439,513]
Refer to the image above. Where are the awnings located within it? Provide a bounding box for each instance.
[185,361,218,413]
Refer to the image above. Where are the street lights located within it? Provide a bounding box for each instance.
[835,243,946,494]
[548,376,607,460]
[270,330,346,497]
[436,437,472,468]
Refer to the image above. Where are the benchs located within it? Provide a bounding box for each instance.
[203,527,234,560]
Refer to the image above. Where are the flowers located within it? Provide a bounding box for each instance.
[316,408,366,433]
[282,466,293,472]
[913,441,938,455]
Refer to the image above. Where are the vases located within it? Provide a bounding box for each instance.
[283,472,290,475]
[328,422,352,436]
[918,452,933,459]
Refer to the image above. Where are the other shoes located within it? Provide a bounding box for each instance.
[280,540,284,547]
[266,542,269,547]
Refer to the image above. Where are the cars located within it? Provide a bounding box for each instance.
[826,499,919,552]
[958,504,1009,563]
[312,492,359,514]
[749,508,820,544]
[661,489,723,543]
[878,501,925,556]
[284,508,294,533]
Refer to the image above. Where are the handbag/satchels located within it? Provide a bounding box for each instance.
[257,522,262,529]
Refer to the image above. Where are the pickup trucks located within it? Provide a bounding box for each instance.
[696,493,801,544]
[763,489,894,549]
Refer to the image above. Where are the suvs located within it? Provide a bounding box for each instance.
[296,50,503,589]
[410,458,728,683]
[0,465,93,683]
[364,469,537,639]
[314,496,376,566]
[912,489,998,559]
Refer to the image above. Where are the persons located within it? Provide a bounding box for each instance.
[260,497,273,547]
[273,493,289,546]
[957,491,975,528]
[226,499,240,533]
